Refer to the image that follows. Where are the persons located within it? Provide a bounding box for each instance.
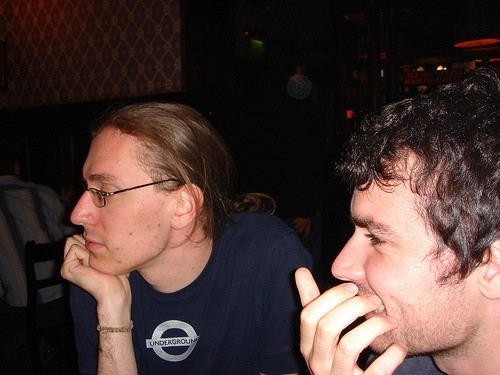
[293,68,500,375]
[0,58,421,375]
[60,103,318,375]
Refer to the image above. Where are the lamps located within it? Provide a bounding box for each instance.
[451,0,500,49]
[286,65,313,99]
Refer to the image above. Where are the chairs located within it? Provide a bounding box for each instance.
[0,238,76,375]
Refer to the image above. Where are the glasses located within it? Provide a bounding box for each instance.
[70,171,181,207]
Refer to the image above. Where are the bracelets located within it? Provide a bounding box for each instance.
[96,321,132,332]
